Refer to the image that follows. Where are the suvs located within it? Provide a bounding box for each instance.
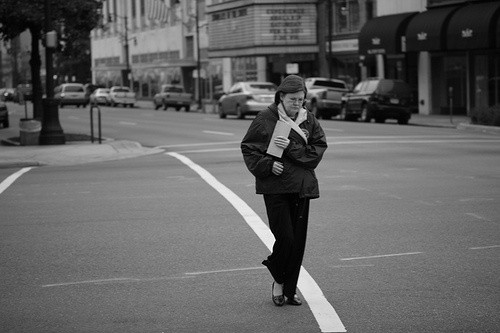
[84,83,106,96]
[53,83,89,108]
[341,77,415,125]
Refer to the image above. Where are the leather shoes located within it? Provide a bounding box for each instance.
[272,282,285,305]
[287,295,302,305]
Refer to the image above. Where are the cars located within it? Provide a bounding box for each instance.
[217,81,278,120]
[89,87,111,107]
[0,83,34,104]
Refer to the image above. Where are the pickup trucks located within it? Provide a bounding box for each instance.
[303,78,352,120]
[153,84,193,112]
[105,86,137,109]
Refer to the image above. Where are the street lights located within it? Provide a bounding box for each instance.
[178,7,209,113]
[106,13,138,90]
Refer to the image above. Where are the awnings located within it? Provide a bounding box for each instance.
[359,12,420,55]
[406,7,460,50]
[446,3,500,50]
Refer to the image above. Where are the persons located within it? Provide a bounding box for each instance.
[240,76,328,306]
